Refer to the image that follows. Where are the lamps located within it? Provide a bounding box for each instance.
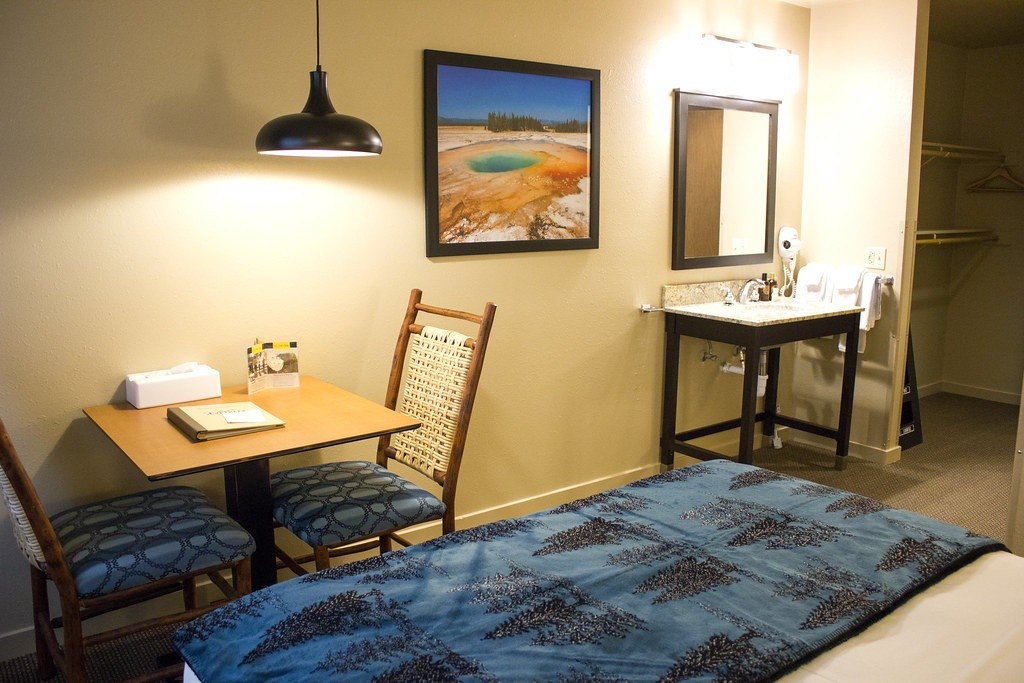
[254,0,385,158]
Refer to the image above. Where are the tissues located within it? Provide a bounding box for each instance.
[125,362,222,409]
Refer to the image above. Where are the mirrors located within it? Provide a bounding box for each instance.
[671,88,782,273]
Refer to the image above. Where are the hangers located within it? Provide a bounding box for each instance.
[965,152,1024,193]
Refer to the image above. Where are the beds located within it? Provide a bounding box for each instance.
[170,459,1022,683]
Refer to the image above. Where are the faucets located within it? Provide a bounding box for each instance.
[737,278,766,304]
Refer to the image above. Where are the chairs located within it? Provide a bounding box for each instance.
[1,419,260,683]
[269,287,497,575]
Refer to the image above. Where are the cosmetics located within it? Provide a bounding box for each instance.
[750,272,779,302]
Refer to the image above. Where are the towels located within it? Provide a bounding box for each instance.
[794,262,883,354]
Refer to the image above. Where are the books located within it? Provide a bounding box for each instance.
[168,401,287,444]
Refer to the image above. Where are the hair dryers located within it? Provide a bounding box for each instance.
[783,239,801,271]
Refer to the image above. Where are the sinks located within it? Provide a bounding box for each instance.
[724,299,801,317]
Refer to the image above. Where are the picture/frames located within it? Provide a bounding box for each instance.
[421,47,600,259]
